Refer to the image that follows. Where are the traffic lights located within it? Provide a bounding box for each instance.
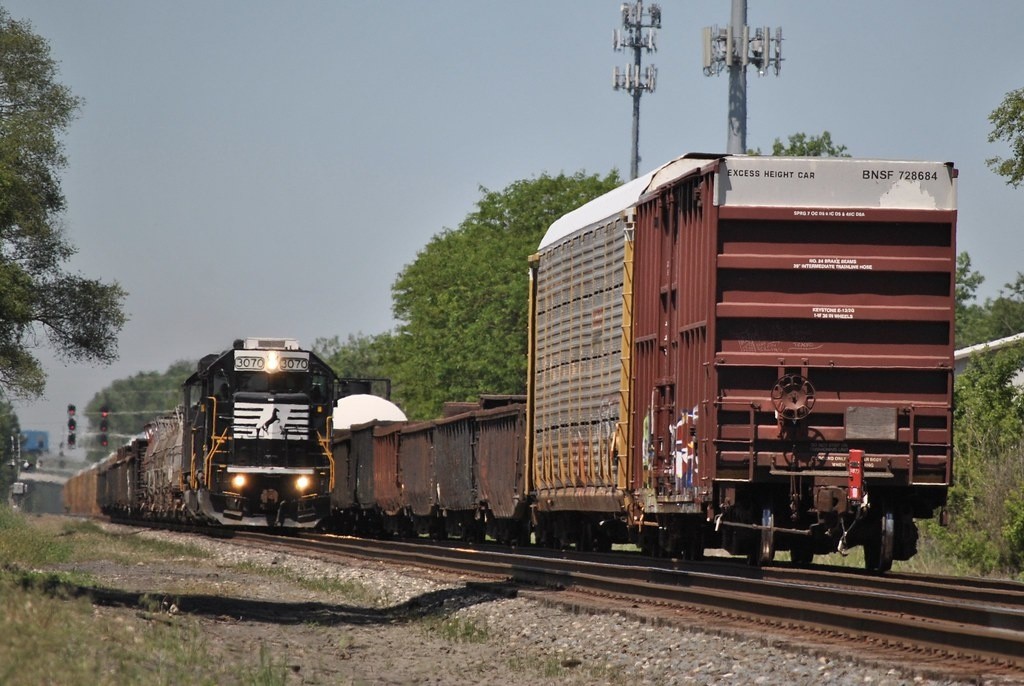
[98,407,110,448]
[66,404,76,447]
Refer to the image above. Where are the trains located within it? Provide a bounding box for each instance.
[60,335,348,535]
[331,150,959,577]
